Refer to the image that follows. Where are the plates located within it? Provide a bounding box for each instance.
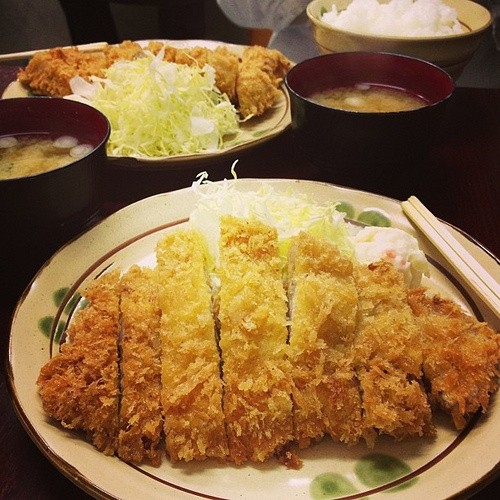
[4,177,500,500]
[2,40,296,171]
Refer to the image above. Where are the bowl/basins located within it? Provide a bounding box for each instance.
[305,0,493,81]
[0,98,110,255]
[285,51,457,188]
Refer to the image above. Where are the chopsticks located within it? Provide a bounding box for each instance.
[402,196,500,317]
[0,42,109,62]
